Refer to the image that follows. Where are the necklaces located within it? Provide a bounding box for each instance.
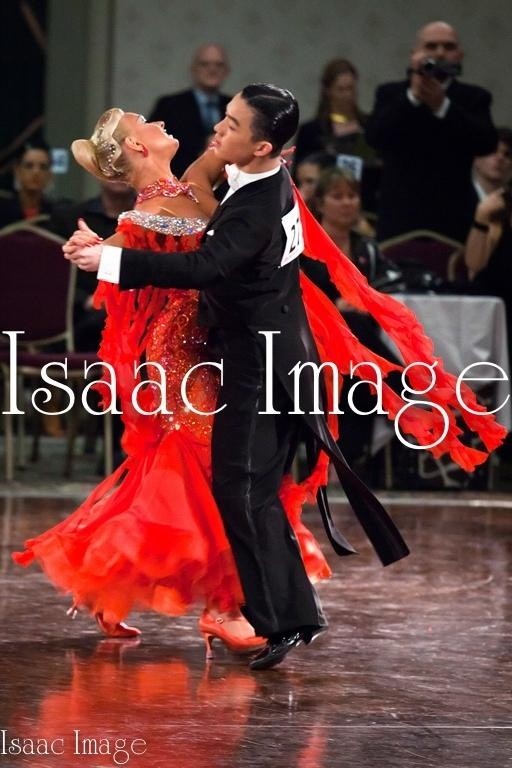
[129,176,205,206]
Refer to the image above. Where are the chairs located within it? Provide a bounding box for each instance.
[377,230,511,490]
[0,222,113,481]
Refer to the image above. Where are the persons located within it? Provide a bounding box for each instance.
[8,106,507,660]
[61,81,413,672]
[2,123,510,492]
[143,43,237,203]
[362,16,497,295]
[292,53,372,176]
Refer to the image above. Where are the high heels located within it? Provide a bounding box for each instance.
[198,609,269,658]
[66,601,143,637]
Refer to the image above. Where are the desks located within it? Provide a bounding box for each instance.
[336,295,512,491]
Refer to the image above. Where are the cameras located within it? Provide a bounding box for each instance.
[418,56,461,80]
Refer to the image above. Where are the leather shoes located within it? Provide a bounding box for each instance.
[250,625,328,670]
